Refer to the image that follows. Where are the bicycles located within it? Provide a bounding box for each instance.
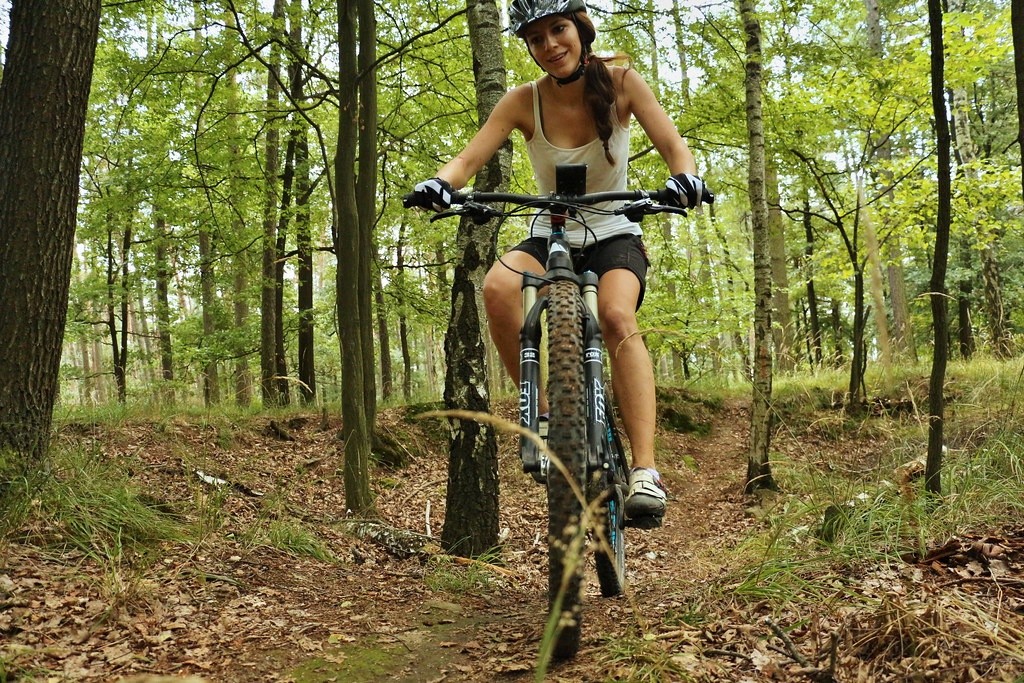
[401,186,715,663]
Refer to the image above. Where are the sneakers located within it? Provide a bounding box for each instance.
[625,467,666,516]
[530,415,550,485]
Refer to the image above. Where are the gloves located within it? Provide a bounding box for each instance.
[414,178,453,214]
[666,173,704,209]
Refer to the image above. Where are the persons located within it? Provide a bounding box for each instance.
[412,1,707,524]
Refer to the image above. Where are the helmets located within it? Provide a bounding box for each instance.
[507,0,588,35]
